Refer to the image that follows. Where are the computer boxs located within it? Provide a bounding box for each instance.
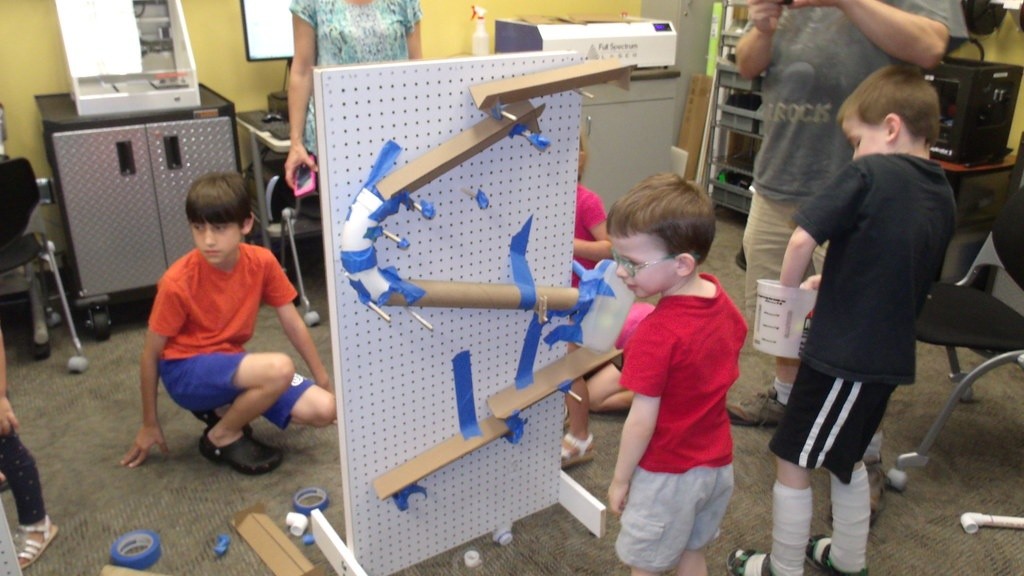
[267,95,290,121]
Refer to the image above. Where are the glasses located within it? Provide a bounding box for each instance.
[611,242,679,277]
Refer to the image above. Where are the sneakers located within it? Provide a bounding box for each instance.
[829,461,886,527]
[726,386,787,427]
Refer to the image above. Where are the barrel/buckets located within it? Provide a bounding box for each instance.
[752,279,818,360]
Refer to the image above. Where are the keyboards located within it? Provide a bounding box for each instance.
[269,122,291,141]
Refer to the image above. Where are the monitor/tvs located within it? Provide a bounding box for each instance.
[240,0,295,62]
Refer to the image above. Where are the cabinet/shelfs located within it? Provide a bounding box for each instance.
[34,83,244,340]
[705,0,765,216]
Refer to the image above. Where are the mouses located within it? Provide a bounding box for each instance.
[261,112,282,122]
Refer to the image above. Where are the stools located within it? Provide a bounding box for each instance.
[266,161,321,326]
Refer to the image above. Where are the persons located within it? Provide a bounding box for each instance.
[726,65,957,576]
[0,325,59,570]
[609,173,749,576]
[120,169,338,476]
[284,0,423,190]
[722,0,968,530]
[562,126,656,468]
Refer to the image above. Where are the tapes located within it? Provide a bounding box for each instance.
[111,530,161,569]
[293,487,329,516]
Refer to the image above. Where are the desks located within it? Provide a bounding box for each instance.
[234,110,322,252]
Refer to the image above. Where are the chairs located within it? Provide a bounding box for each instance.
[887,186,1024,491]
[0,104,89,373]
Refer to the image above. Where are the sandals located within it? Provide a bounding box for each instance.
[559,434,594,469]
[805,534,869,576]
[12,514,59,571]
[193,406,253,438]
[199,425,283,475]
[726,547,773,576]
